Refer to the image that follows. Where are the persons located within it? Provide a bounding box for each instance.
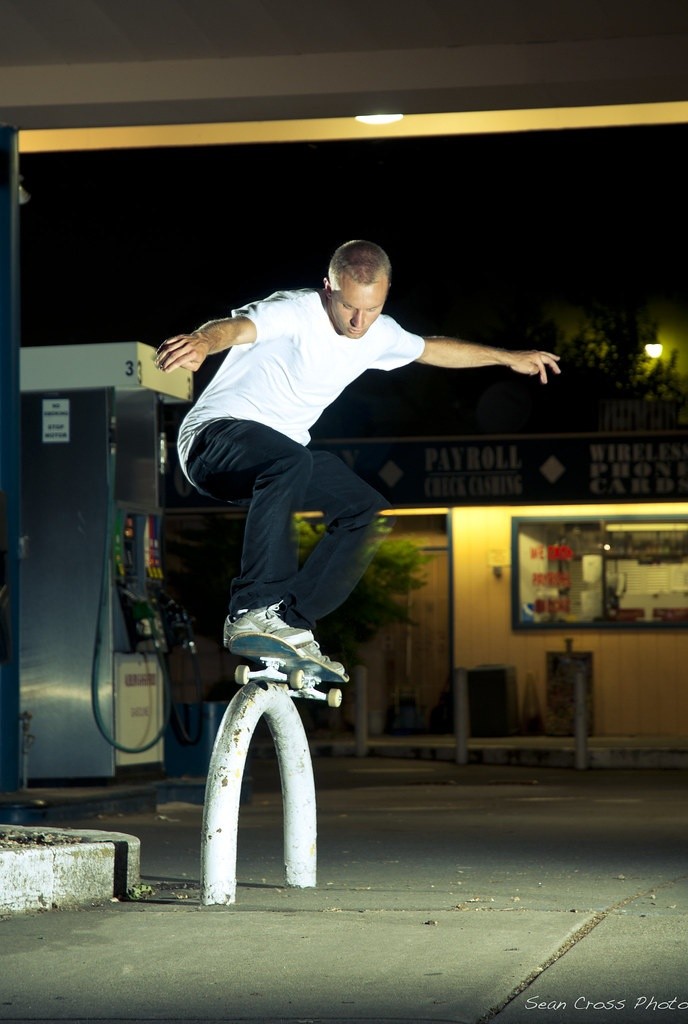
[155,237,564,674]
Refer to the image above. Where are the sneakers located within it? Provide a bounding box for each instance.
[293,640,345,676]
[224,608,313,649]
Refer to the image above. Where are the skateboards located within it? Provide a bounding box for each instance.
[228,631,350,708]
